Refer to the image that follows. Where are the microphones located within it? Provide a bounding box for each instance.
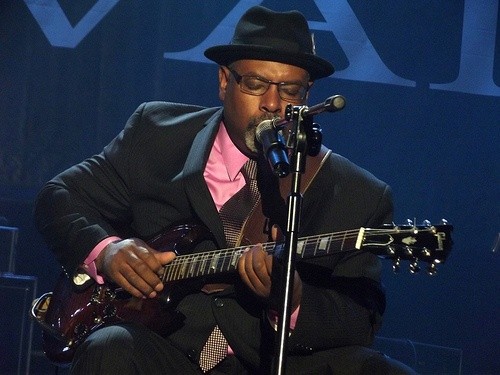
[256,120,291,178]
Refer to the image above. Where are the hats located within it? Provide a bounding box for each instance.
[203,6,335,81]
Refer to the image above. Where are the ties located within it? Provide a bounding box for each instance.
[194,158,266,371]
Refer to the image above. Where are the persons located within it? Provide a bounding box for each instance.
[34,6,417,375]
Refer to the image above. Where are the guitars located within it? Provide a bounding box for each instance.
[31,217,454,365]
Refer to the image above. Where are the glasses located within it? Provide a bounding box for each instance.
[230,68,309,101]
